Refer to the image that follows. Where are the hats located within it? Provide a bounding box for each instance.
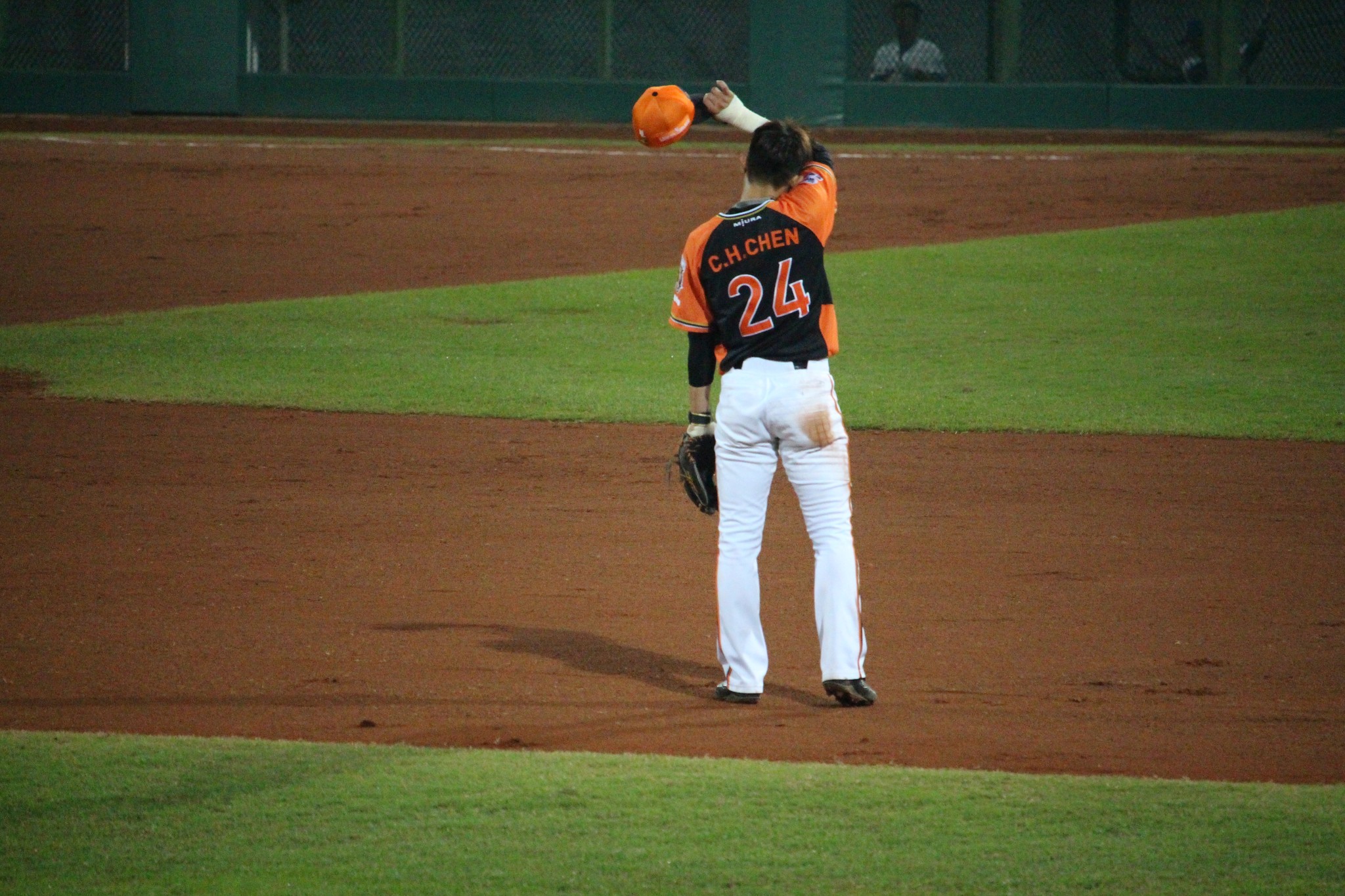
[632,85,713,147]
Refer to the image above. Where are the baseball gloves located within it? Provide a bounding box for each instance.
[678,414,720,515]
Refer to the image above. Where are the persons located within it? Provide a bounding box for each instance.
[668,79,882,703]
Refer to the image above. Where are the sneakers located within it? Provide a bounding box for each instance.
[823,678,876,708]
[714,681,760,703]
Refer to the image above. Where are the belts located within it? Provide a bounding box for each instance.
[733,357,808,370]
